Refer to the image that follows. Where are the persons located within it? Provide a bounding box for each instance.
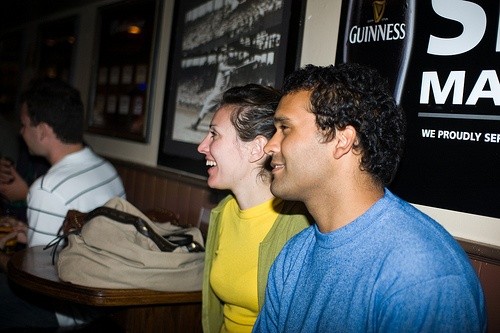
[5,76,128,248]
[251,62,489,333]
[0,156,29,202]
[198,82,315,333]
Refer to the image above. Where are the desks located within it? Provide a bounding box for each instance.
[5,242,204,312]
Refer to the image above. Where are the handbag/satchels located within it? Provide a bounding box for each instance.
[55,198,206,294]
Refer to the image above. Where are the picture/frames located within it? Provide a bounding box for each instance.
[31,12,82,90]
[334,0,500,219]
[155,0,307,179]
[79,0,167,145]
